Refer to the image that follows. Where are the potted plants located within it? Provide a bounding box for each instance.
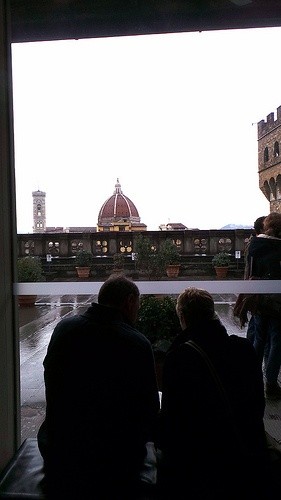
[17,257,42,305]
[137,296,180,390]
[213,252,231,279]
[75,252,91,278]
[164,245,182,277]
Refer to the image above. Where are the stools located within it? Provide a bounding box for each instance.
[0,439,157,500]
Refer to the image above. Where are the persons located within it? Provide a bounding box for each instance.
[232,213,281,399]
[38,274,161,500]
[155,289,281,500]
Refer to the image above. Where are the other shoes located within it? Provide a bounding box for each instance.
[266,385,281,399]
